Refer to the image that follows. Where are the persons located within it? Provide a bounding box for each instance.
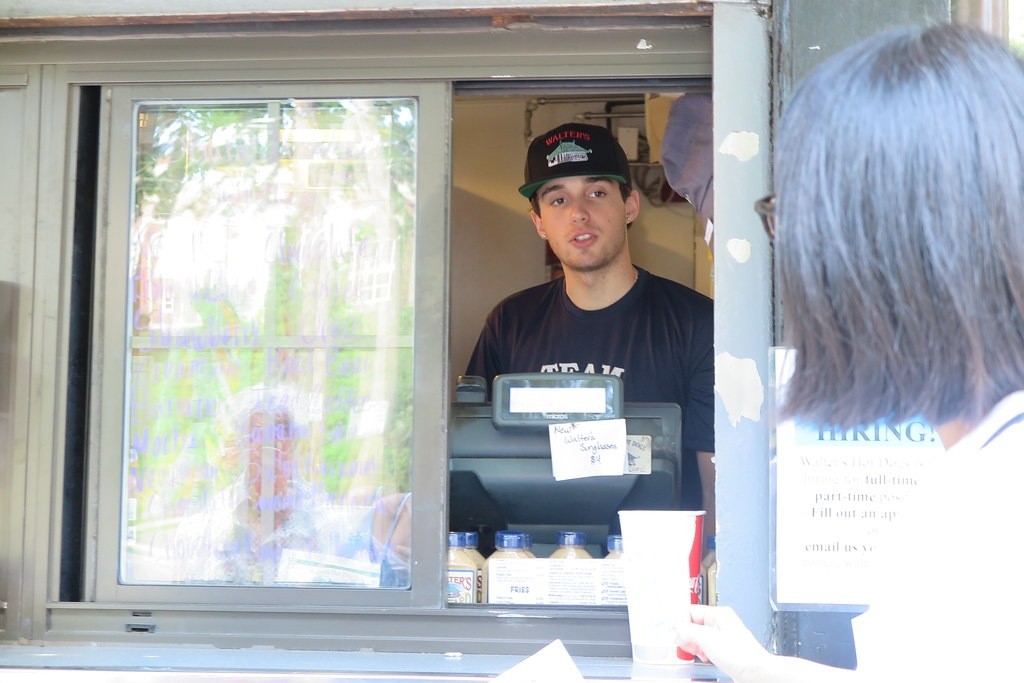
[673,21,1024,683]
[446,123,715,555]
[196,399,368,584]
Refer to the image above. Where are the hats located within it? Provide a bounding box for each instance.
[518,123,632,196]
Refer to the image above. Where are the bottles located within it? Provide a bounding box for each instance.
[549,530,592,558]
[603,535,623,559]
[482,529,535,603]
[700,536,716,606]
[447,530,486,605]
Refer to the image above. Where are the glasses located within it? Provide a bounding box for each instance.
[754,191,776,236]
